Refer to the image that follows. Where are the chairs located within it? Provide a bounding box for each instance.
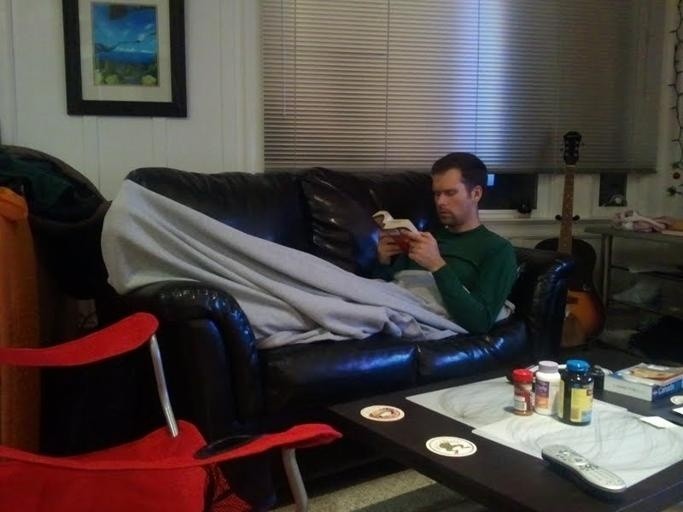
[0,309,346,512]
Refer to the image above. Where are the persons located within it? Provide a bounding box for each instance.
[376,153,518,341]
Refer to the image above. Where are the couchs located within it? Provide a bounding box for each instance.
[100,164,574,510]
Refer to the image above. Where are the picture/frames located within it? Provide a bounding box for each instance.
[59,0,191,122]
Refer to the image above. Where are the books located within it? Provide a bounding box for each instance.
[603,361,681,403]
[372,209,420,256]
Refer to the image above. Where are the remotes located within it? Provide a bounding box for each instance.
[541,444,627,493]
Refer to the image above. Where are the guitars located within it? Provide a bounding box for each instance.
[533,129,607,351]
